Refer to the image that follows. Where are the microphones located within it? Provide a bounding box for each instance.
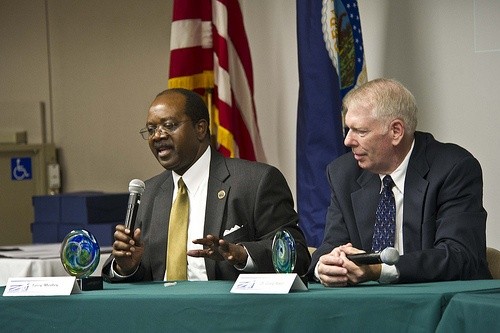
[346,247,400,266]
[124,179,145,238]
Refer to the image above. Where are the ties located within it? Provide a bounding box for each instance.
[166,177,189,281]
[371,174,396,254]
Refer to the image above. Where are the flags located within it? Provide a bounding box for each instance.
[168,0,268,165]
[296,0,369,249]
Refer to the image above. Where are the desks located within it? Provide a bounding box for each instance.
[0,243,500,333]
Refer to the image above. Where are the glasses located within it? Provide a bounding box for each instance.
[139,119,196,140]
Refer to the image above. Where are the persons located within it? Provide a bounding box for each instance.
[100,88,312,285]
[301,78,488,287]
